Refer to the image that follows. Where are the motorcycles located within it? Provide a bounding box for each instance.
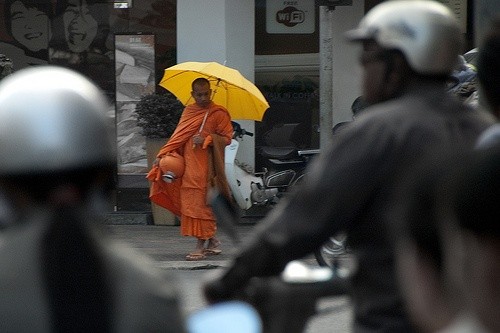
[224,121,322,222]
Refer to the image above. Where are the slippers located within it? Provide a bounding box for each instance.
[185,252,208,261]
[204,249,221,256]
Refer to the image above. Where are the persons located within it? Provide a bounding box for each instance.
[201,0,499,333]
[0,66,181,333]
[157,77,232,261]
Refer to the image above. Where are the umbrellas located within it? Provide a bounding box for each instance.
[159,60,271,149]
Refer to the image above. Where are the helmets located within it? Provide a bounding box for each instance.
[0,66,116,176]
[347,0,467,75]
